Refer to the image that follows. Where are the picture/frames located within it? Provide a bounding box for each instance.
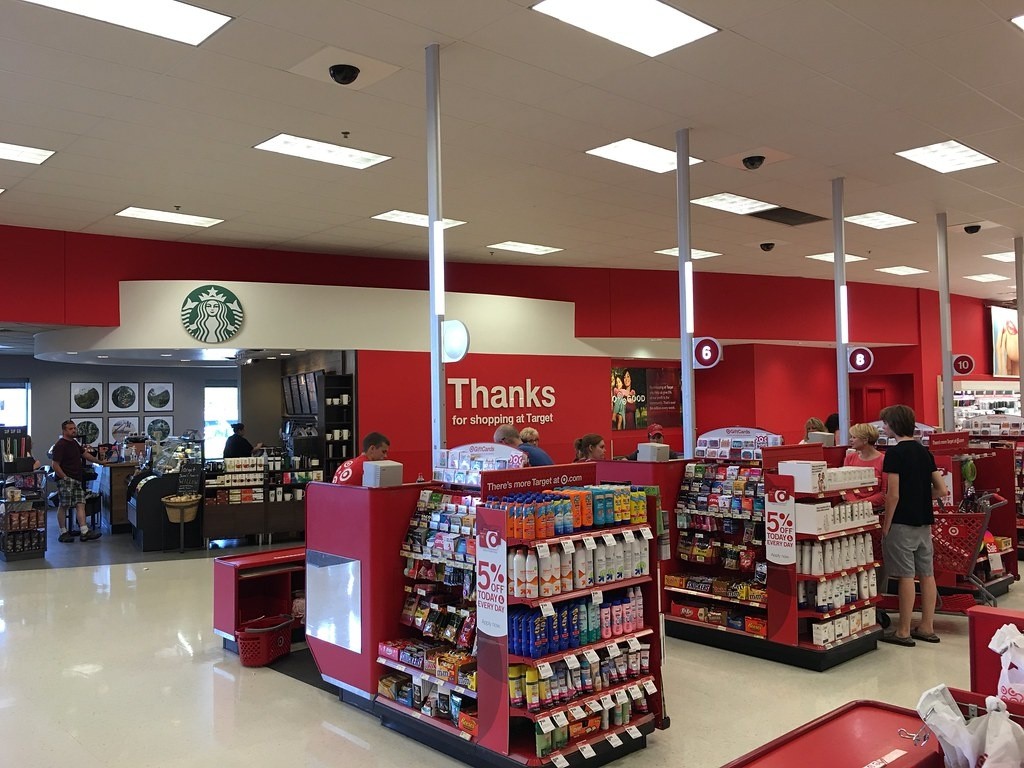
[143,382,174,412]
[70,382,103,413]
[144,415,174,436]
[108,382,140,413]
[70,417,103,447]
[108,416,140,444]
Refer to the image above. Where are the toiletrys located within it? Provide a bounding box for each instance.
[550,640,650,707]
[798,567,877,613]
[502,586,644,659]
[507,532,650,599]
[795,531,875,575]
[260,446,290,471]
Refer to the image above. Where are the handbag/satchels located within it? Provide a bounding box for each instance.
[997,641,1024,705]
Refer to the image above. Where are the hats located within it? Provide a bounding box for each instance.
[647,423,664,438]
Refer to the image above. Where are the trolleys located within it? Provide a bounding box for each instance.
[875,492,1009,629]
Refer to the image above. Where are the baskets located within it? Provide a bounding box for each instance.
[235,613,295,667]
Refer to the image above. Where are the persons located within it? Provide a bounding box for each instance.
[491,424,555,466]
[881,405,948,647]
[798,413,840,446]
[611,369,638,429]
[331,432,391,487]
[52,419,111,543]
[842,423,888,514]
[573,433,607,462]
[621,423,679,461]
[222,423,264,461]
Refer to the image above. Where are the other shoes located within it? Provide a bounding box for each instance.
[80,530,102,542]
[910,627,940,643]
[59,532,75,543]
[877,630,916,647]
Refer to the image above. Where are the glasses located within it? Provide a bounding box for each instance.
[527,439,539,444]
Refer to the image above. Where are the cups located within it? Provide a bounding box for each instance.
[325,428,352,441]
[325,394,352,406]
[269,487,306,503]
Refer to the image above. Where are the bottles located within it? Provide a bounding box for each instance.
[484,484,650,759]
[138,451,144,465]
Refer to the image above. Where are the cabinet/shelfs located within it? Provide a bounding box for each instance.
[662,585,767,660]
[506,523,657,768]
[0,470,47,562]
[373,656,478,766]
[318,373,354,484]
[794,484,883,672]
